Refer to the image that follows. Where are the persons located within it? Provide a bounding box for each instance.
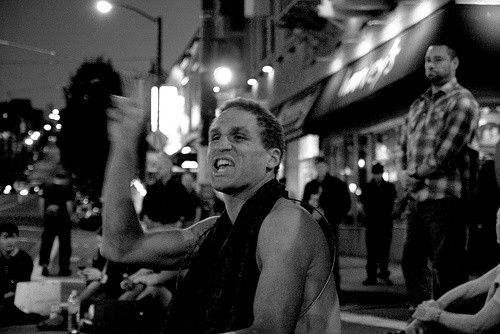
[38,170,73,280]
[399,39,481,316]
[0,221,34,329]
[99,74,342,334]
[299,156,353,293]
[37,145,226,334]
[356,162,401,288]
[404,207,500,334]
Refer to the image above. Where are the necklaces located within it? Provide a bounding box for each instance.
[436,309,444,322]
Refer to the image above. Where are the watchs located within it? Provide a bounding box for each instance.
[408,165,426,181]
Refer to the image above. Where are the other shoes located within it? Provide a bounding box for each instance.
[58,268,72,276]
[363,277,378,285]
[42,267,49,275]
[379,277,395,285]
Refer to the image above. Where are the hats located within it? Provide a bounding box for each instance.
[372,162,385,173]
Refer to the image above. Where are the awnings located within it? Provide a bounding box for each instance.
[298,0,459,141]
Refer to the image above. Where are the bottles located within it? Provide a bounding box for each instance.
[49,302,58,319]
[67,289,80,334]
